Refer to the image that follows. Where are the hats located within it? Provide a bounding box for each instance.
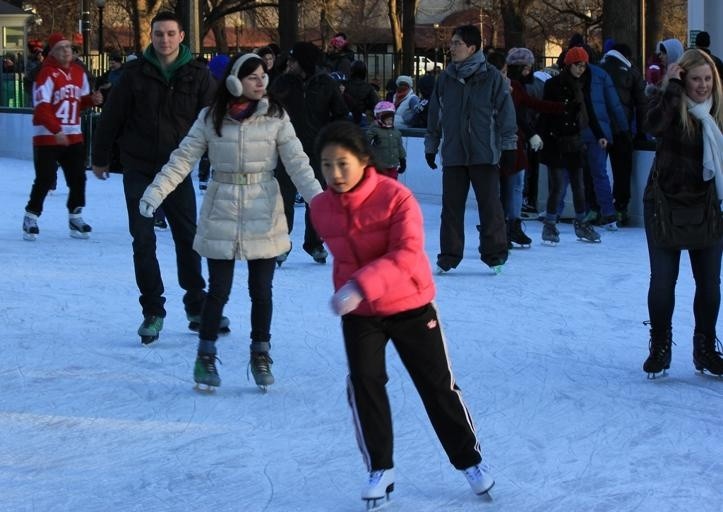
[210,57,230,79]
[396,76,413,88]
[48,32,66,47]
[564,48,589,65]
[507,47,535,67]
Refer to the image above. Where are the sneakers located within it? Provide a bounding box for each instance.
[521,205,538,214]
[437,258,451,272]
[573,222,600,239]
[601,215,617,225]
[643,325,673,373]
[279,243,293,261]
[154,220,168,229]
[304,244,329,258]
[137,314,162,336]
[487,257,505,266]
[360,469,394,499]
[543,225,560,243]
[187,313,230,327]
[194,355,221,386]
[199,180,208,190]
[506,219,532,244]
[294,192,306,205]
[249,353,275,384]
[463,461,496,494]
[69,218,91,231]
[693,334,722,376]
[21,219,40,233]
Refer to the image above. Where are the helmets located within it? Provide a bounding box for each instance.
[327,72,346,83]
[374,102,395,116]
[331,37,346,50]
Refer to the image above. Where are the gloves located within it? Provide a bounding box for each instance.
[529,135,544,152]
[330,281,364,314]
[397,157,408,173]
[424,152,437,169]
[139,202,156,217]
[500,150,514,173]
[648,66,661,86]
[620,131,633,144]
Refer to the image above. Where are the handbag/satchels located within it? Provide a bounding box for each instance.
[649,143,721,250]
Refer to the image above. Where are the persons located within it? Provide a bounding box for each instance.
[258,48,277,91]
[25,41,43,96]
[393,74,424,131]
[537,42,631,231]
[92,12,230,345]
[487,51,531,250]
[2,52,23,75]
[98,55,122,85]
[426,24,517,275]
[310,121,496,510]
[364,101,407,181]
[138,51,325,395]
[418,66,441,92]
[277,42,347,269]
[22,30,102,244]
[349,60,379,110]
[696,32,723,79]
[508,47,544,222]
[644,47,723,380]
[330,70,347,96]
[529,47,610,246]
[71,31,95,95]
[325,33,354,70]
[644,38,685,98]
